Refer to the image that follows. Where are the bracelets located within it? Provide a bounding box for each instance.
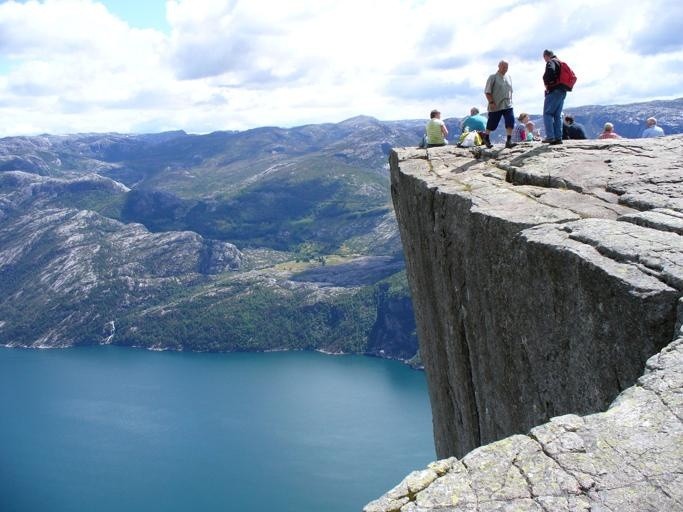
[489,101,494,105]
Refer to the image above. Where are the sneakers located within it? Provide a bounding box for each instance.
[485,138,493,147]
[506,141,516,148]
[542,138,563,143]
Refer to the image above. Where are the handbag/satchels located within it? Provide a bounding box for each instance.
[559,63,577,91]
[460,131,486,146]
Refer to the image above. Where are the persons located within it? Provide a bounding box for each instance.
[459,108,487,132]
[642,117,664,138]
[596,123,621,139]
[551,112,570,140]
[418,110,449,150]
[524,121,535,141]
[484,60,518,149]
[563,115,588,139]
[541,50,566,145]
[514,112,529,141]
